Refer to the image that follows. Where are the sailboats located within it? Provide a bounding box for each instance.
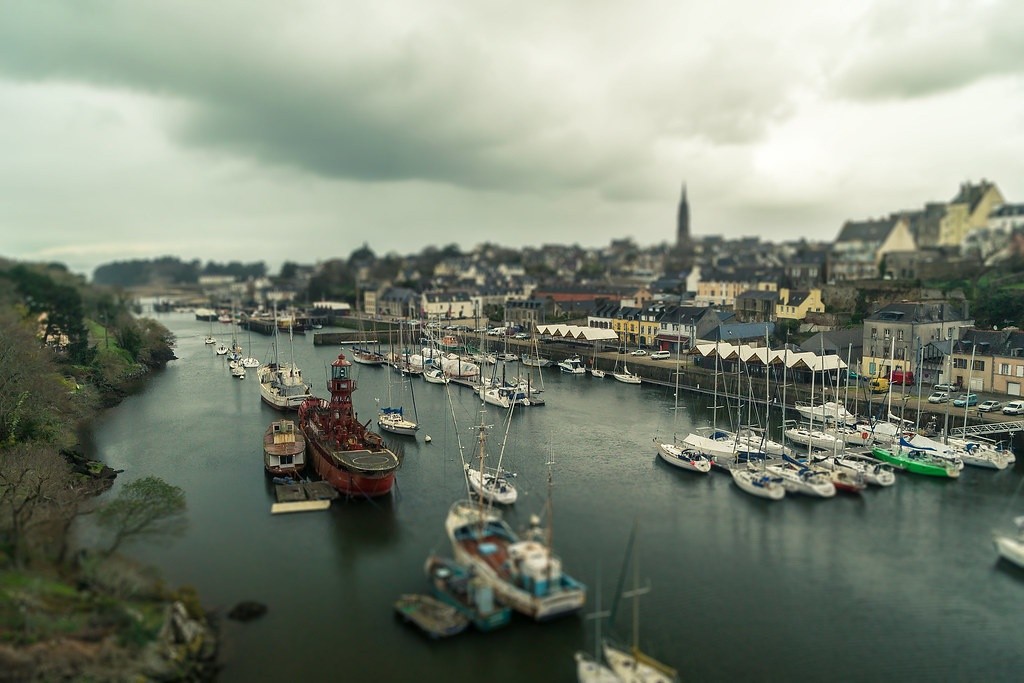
[202,288,589,643]
[590,339,606,379]
[990,475,1024,573]
[573,559,629,683]
[603,554,682,683]
[611,323,642,384]
[649,309,1017,501]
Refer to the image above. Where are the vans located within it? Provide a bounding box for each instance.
[1002,400,1024,416]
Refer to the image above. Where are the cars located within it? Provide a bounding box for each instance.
[928,391,950,404]
[977,400,1001,412]
[843,369,857,380]
[630,349,647,357]
[933,382,961,392]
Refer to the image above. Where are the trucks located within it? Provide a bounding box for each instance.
[882,370,914,386]
[869,378,890,394]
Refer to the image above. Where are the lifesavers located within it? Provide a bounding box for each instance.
[862,432,868,439]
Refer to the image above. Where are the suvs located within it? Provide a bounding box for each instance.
[650,350,671,360]
[953,393,978,407]
